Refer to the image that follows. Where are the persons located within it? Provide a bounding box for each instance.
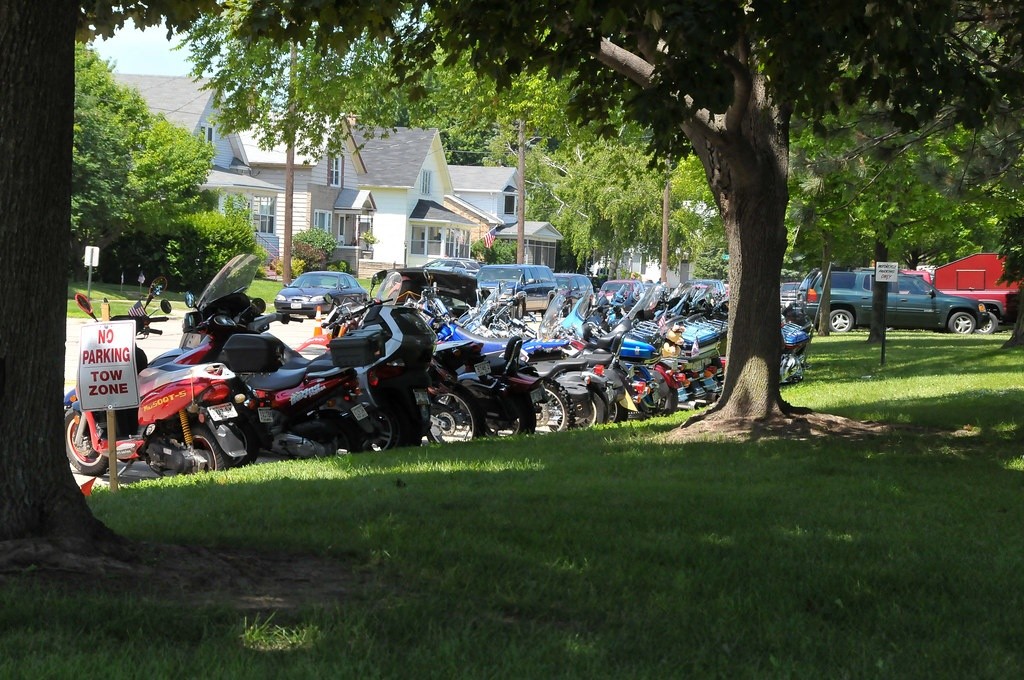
[630,272,642,282]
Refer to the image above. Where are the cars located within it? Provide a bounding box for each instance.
[273,270,369,324]
[424,257,800,316]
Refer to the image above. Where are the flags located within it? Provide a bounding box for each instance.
[658,308,667,336]
[137,273,145,283]
[128,302,147,318]
[484,229,496,248]
[691,340,699,356]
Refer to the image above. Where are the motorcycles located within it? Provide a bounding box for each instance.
[177,253,387,460]
[299,267,812,452]
[63,276,255,479]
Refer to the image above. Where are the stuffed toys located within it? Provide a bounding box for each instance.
[662,325,686,357]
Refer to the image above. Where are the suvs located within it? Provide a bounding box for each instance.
[794,271,991,335]
